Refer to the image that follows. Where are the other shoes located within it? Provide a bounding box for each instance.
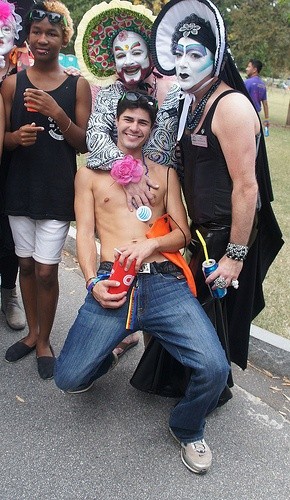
[0,283,26,331]
[34,341,57,381]
[5,336,45,365]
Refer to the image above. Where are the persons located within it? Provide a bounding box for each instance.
[243,59,270,128]
[64,0,185,358]
[0,0,90,381]
[130,0,285,417]
[54,89,230,476]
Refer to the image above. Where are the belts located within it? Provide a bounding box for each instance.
[98,261,181,275]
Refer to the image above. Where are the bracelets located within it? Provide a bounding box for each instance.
[110,154,145,186]
[62,118,71,135]
[85,277,101,295]
[263,117,269,122]
[226,243,249,261]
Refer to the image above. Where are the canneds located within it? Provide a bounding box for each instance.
[202,259,227,299]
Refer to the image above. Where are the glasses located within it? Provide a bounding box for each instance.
[120,91,157,110]
[30,9,61,24]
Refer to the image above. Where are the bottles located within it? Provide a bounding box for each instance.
[264,126,269,137]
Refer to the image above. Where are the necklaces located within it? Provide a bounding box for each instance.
[120,151,154,222]
[124,76,158,98]
[185,79,223,131]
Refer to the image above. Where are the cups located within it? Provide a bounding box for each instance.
[107,254,143,302]
[25,89,43,112]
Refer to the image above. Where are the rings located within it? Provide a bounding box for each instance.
[231,279,239,289]
[214,278,227,288]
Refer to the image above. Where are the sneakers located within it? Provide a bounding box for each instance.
[64,350,120,397]
[169,421,214,473]
[113,333,138,357]
